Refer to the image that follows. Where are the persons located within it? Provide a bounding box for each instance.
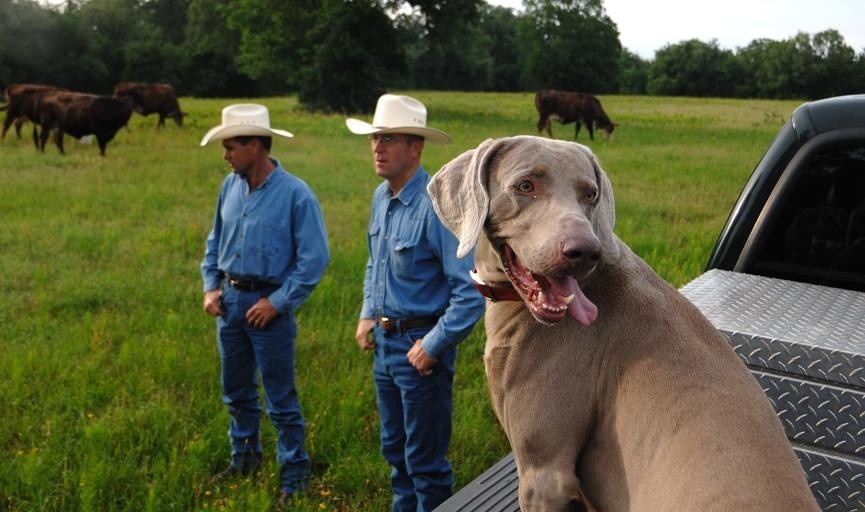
[195,99,331,499]
[338,87,484,509]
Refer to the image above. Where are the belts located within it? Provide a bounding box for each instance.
[225,274,270,290]
[376,314,437,333]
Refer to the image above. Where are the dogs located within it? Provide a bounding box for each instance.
[427,136,823,512]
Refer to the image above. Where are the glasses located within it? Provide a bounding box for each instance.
[367,135,415,146]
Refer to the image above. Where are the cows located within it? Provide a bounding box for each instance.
[112,82,189,134]
[33,88,143,157]
[535,88,621,141]
[0,84,69,144]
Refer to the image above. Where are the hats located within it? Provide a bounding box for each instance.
[345,93,451,144]
[200,103,294,147]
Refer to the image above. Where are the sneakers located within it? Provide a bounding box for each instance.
[213,466,264,484]
[273,493,293,512]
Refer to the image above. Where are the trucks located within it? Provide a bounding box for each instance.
[422,88,863,510]
